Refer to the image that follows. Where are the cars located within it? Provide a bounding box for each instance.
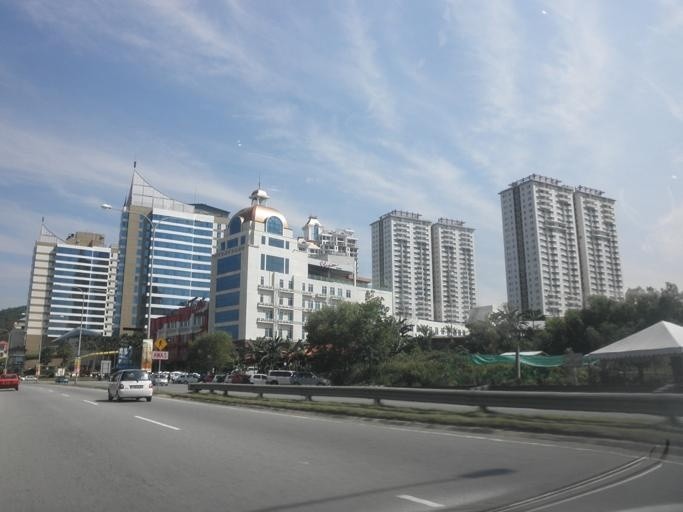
[0,365,55,390]
[108,369,153,402]
[212,366,331,386]
[150,371,200,386]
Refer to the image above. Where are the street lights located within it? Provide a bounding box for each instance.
[509,328,526,379]
[72,204,181,369]
